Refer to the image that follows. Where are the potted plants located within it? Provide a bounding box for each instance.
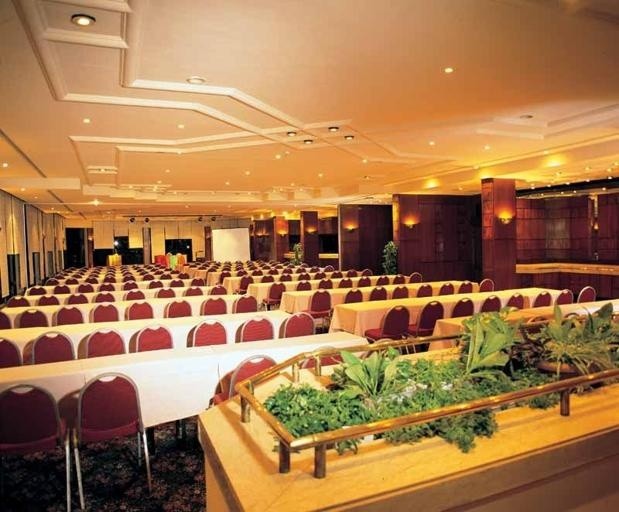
[534,310,617,394]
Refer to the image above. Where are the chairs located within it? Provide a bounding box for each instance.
[1,261,373,371]
[280,272,596,350]
[72,373,155,512]
[215,338,400,403]
[1,385,71,512]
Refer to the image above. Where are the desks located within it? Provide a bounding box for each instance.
[0,332,371,439]
